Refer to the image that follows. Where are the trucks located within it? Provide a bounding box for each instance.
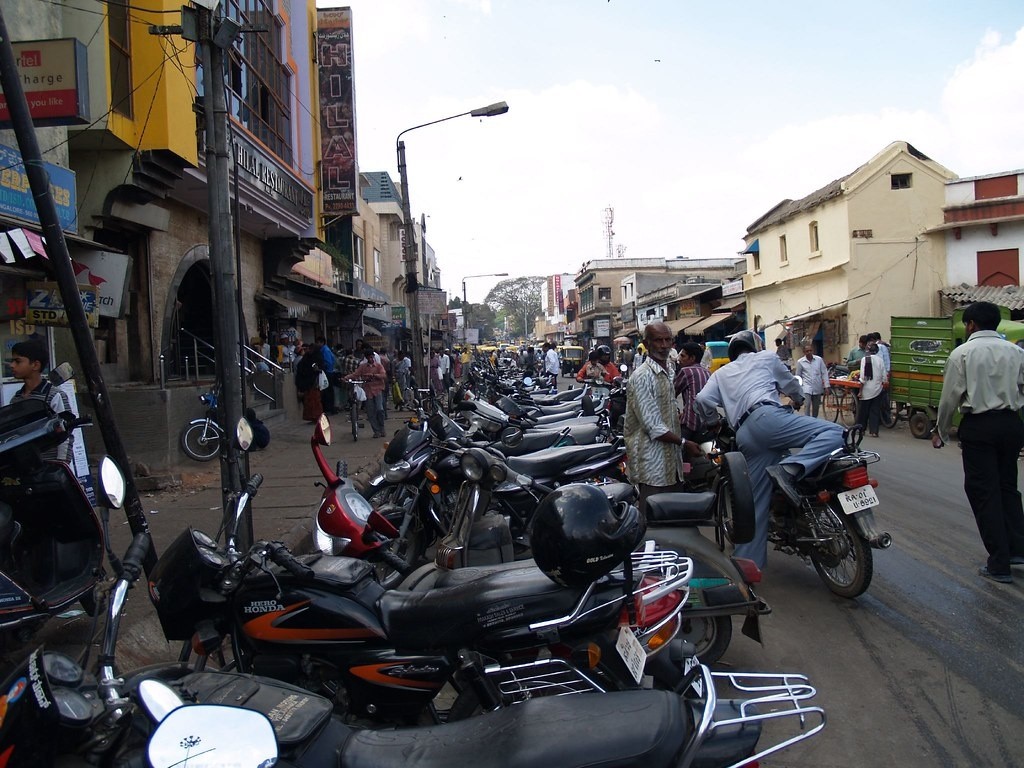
[888,304,1024,439]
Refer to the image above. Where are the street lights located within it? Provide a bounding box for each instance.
[462,273,508,329]
[395,101,510,390]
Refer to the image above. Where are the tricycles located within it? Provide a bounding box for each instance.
[822,363,899,430]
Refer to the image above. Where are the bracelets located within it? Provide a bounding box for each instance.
[679,437,686,450]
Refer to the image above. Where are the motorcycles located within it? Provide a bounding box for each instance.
[561,346,584,378]
[698,403,893,599]
[180,386,225,461]
[0,342,828,768]
[704,342,731,377]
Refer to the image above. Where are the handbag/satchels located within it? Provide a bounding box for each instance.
[354,384,367,401]
[392,382,403,404]
[437,366,443,380]
[318,371,329,391]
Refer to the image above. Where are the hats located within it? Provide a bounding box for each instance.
[280,333,289,339]
[294,345,306,354]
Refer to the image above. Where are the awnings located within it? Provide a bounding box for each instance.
[724,290,871,352]
[613,327,638,337]
[263,289,311,319]
[684,309,734,336]
[666,316,705,338]
[286,276,389,332]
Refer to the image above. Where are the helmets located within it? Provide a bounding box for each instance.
[728,330,766,362]
[597,345,611,365]
[529,483,648,587]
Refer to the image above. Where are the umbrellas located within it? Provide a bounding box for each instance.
[614,336,632,345]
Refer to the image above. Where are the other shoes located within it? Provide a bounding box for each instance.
[872,433,878,437]
[381,431,386,436]
[1010,556,1024,564]
[373,432,381,438]
[978,567,1012,582]
[856,430,865,435]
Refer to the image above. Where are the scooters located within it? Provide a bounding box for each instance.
[0,362,108,653]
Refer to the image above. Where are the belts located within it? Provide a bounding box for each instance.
[735,402,773,431]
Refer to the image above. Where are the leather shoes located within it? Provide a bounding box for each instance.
[765,464,802,508]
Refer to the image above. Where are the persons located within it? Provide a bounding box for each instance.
[854,343,889,438]
[7,336,75,468]
[699,343,713,370]
[623,321,706,516]
[576,344,628,443]
[667,342,680,367]
[674,342,712,439]
[848,331,893,425]
[928,301,1024,583]
[695,338,848,572]
[631,346,643,371]
[796,344,830,417]
[612,346,636,379]
[252,327,561,438]
